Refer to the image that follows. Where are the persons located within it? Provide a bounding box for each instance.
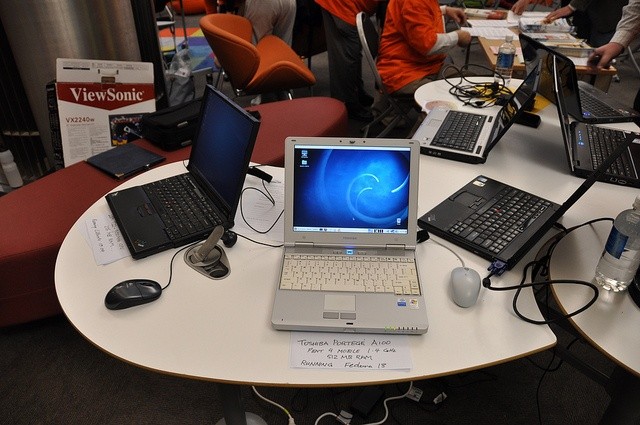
[586,0,640,127]
[315,0,391,124]
[511,0,629,85]
[242,0,297,106]
[375,0,472,139]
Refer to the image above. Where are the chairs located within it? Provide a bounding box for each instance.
[153,0,177,55]
[355,12,413,137]
[200,13,316,99]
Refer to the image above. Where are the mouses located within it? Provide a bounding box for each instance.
[451,266,481,309]
[104,279,162,310]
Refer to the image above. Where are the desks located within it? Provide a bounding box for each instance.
[54,77,640,389]
[465,8,617,93]
[548,220,640,376]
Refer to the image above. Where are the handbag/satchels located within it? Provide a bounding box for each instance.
[143,96,205,152]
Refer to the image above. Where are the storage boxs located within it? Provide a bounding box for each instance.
[55,57,157,168]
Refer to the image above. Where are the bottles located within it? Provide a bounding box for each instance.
[494,34,515,85]
[594,196,639,293]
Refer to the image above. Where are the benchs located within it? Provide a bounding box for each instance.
[0,97,348,329]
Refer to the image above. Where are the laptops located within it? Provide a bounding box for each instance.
[552,54,640,189]
[270,135,428,336]
[85,142,167,183]
[105,84,262,260]
[417,131,640,271]
[519,32,640,124]
[412,59,544,164]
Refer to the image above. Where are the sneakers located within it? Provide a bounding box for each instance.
[358,94,374,107]
[346,101,374,123]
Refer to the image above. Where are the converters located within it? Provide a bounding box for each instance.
[432,392,447,404]
[335,409,354,425]
[352,385,385,416]
